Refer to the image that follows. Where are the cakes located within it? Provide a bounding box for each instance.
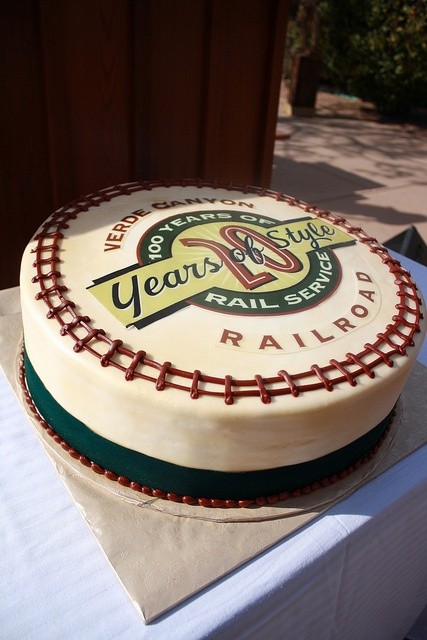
[17,175,425,524]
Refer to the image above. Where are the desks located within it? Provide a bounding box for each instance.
[0,244,427,639]
